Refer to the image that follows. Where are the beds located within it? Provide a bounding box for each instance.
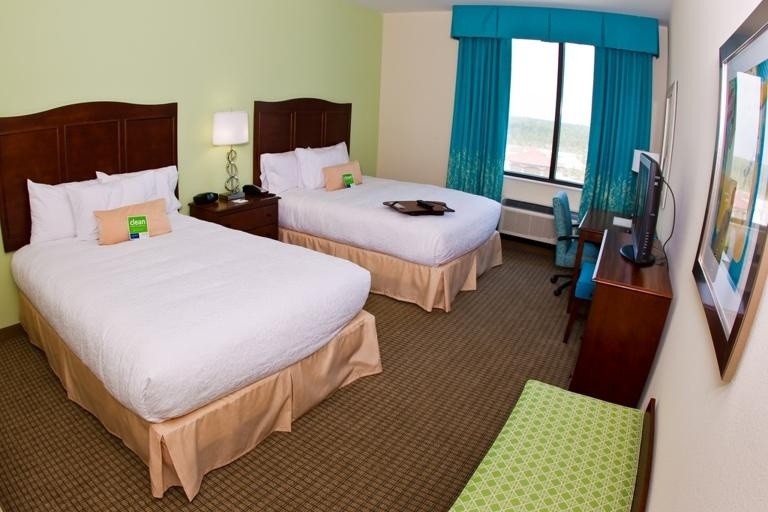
[0,102,384,500]
[253,98,503,312]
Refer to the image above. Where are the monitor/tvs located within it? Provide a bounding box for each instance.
[620,152,664,264]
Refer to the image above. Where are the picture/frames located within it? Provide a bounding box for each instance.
[658,81,679,209]
[692,0,768,384]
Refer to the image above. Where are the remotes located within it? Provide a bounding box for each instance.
[417,200,435,208]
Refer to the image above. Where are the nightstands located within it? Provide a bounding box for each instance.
[187,195,282,242]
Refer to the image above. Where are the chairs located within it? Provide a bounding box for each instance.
[551,191,596,295]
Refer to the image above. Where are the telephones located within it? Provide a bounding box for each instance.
[242,184,275,197]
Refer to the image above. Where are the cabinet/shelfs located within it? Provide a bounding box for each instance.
[568,230,673,410]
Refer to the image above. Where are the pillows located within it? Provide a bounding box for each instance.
[96,166,179,191]
[259,147,310,196]
[296,141,349,191]
[69,171,182,240]
[323,161,363,191]
[92,198,172,246]
[25,177,95,245]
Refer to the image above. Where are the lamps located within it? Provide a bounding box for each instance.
[213,110,249,201]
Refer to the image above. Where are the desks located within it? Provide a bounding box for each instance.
[563,208,635,343]
[447,378,655,511]
